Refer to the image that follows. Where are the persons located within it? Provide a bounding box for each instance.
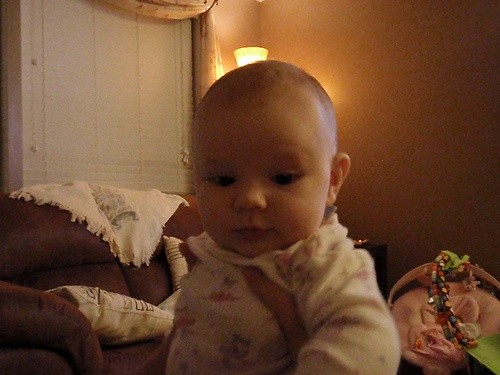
[130,241,310,375]
[159,58,403,375]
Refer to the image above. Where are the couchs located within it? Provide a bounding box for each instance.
[0,193,205,375]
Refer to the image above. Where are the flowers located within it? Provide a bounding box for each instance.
[408,249,482,375]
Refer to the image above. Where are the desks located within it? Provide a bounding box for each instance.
[357,243,387,300]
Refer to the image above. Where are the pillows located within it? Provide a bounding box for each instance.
[46,235,190,350]
